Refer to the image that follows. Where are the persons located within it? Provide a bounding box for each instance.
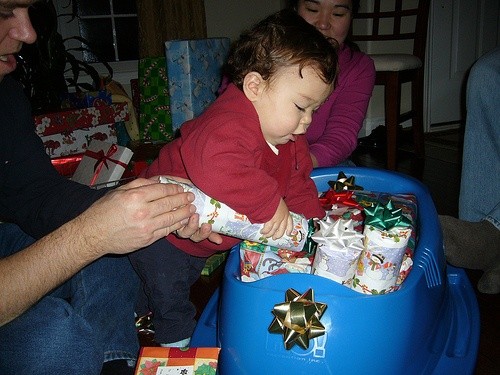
[128,8,326,347]
[217,0,375,168]
[437,201,500,270]
[0,0,222,375]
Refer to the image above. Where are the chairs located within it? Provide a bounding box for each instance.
[348,0,430,171]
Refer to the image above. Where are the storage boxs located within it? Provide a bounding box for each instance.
[32,90,134,189]
[131,38,231,140]
